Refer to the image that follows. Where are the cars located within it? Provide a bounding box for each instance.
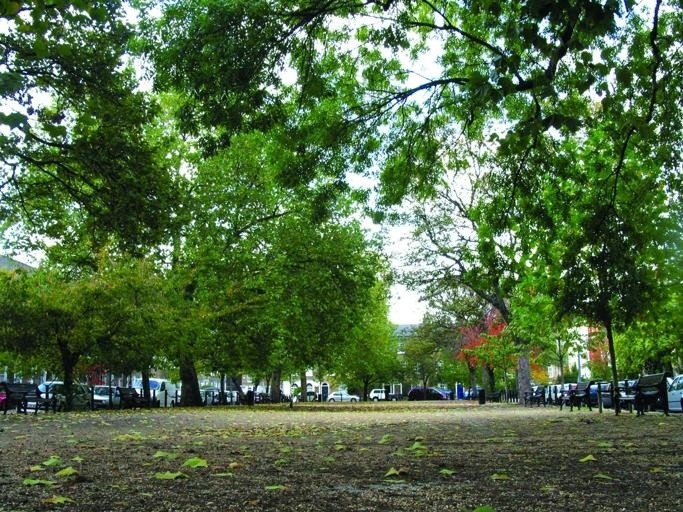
[531,379,637,409]
[325,391,360,403]
[18,378,94,413]
[667,374,683,412]
[464,386,482,400]
[175,388,317,407]
[407,384,452,401]
[91,383,120,409]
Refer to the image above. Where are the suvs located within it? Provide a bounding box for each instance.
[370,388,402,402]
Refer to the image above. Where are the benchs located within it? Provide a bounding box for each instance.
[480,372,672,420]
[0,377,293,416]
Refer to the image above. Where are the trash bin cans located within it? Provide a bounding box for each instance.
[479,390,485,404]
[247,391,254,405]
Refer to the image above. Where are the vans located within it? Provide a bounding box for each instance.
[129,378,175,407]
